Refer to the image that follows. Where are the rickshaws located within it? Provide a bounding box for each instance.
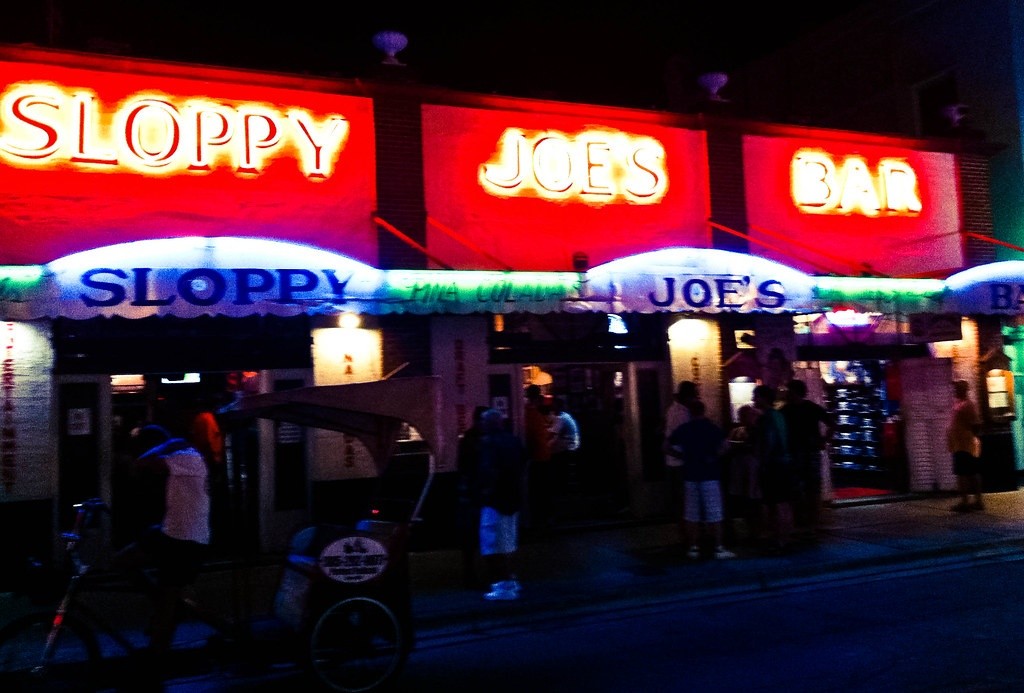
[0,376,441,693]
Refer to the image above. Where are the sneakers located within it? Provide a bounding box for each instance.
[713,545,740,560]
[687,546,700,557]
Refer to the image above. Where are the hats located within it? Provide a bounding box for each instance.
[952,379,970,389]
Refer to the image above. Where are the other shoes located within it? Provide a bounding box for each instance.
[950,502,969,512]
[968,500,985,510]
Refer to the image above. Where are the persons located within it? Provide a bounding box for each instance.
[940,379,988,510]
[459,409,525,602]
[662,378,839,562]
[95,422,213,618]
[520,382,616,526]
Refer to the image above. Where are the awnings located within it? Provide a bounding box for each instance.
[2,233,1024,314]
